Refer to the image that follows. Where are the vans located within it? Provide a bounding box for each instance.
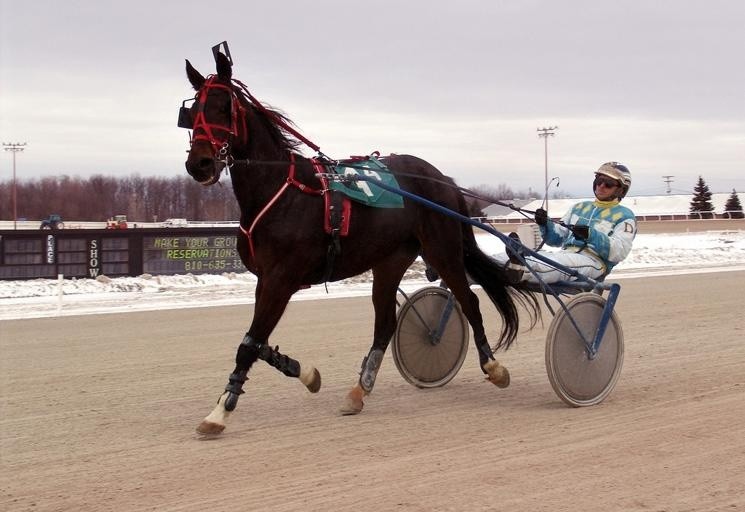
[159,218,187,228]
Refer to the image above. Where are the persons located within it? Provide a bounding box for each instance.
[425,161,638,292]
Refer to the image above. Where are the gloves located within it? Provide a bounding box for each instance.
[572,225,589,241]
[535,209,547,225]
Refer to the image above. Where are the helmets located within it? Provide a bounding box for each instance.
[594,161,631,198]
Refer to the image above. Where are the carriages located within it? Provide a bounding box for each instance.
[178,41,625,436]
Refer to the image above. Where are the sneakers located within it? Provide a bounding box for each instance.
[505,232,525,284]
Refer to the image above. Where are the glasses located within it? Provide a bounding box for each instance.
[596,177,619,188]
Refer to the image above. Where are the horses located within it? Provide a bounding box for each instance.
[185,50,544,436]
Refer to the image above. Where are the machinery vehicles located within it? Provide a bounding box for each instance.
[40,214,64,229]
[106,215,128,229]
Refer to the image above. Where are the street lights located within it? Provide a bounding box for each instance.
[2,142,27,230]
[535,125,560,214]
[661,175,675,195]
